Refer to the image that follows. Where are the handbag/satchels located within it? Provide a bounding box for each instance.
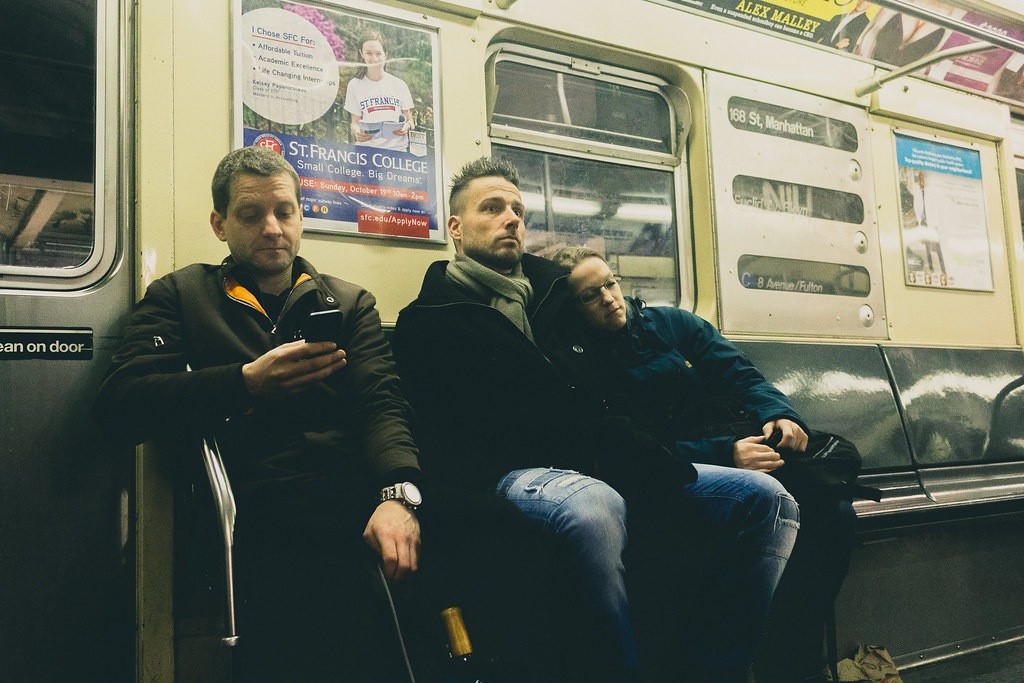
[764,430,860,484]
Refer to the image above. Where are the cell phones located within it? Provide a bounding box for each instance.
[305,309,344,350]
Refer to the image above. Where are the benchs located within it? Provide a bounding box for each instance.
[175,340,1024,651]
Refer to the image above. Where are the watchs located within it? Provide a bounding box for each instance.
[379,482,423,510]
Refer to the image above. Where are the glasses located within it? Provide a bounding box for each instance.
[577,275,622,304]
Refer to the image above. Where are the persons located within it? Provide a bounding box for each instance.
[544,244,865,683]
[342,31,416,153]
[391,156,804,681]
[109,147,448,683]
[816,0,872,52]
[854,0,954,74]
